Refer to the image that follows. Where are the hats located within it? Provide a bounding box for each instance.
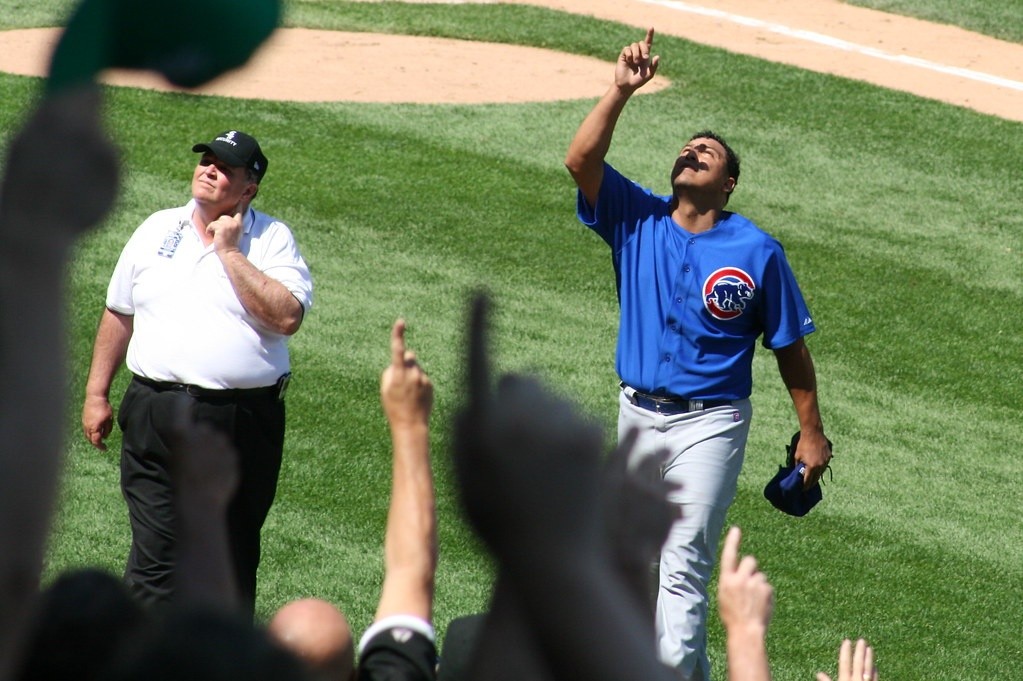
[765,431,832,517]
[192,130,268,182]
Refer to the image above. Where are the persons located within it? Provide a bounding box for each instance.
[82,131,312,621]
[563,27,832,681]
[0,0,874,681]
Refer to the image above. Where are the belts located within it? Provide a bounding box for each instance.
[620,380,732,416]
[132,372,281,399]
